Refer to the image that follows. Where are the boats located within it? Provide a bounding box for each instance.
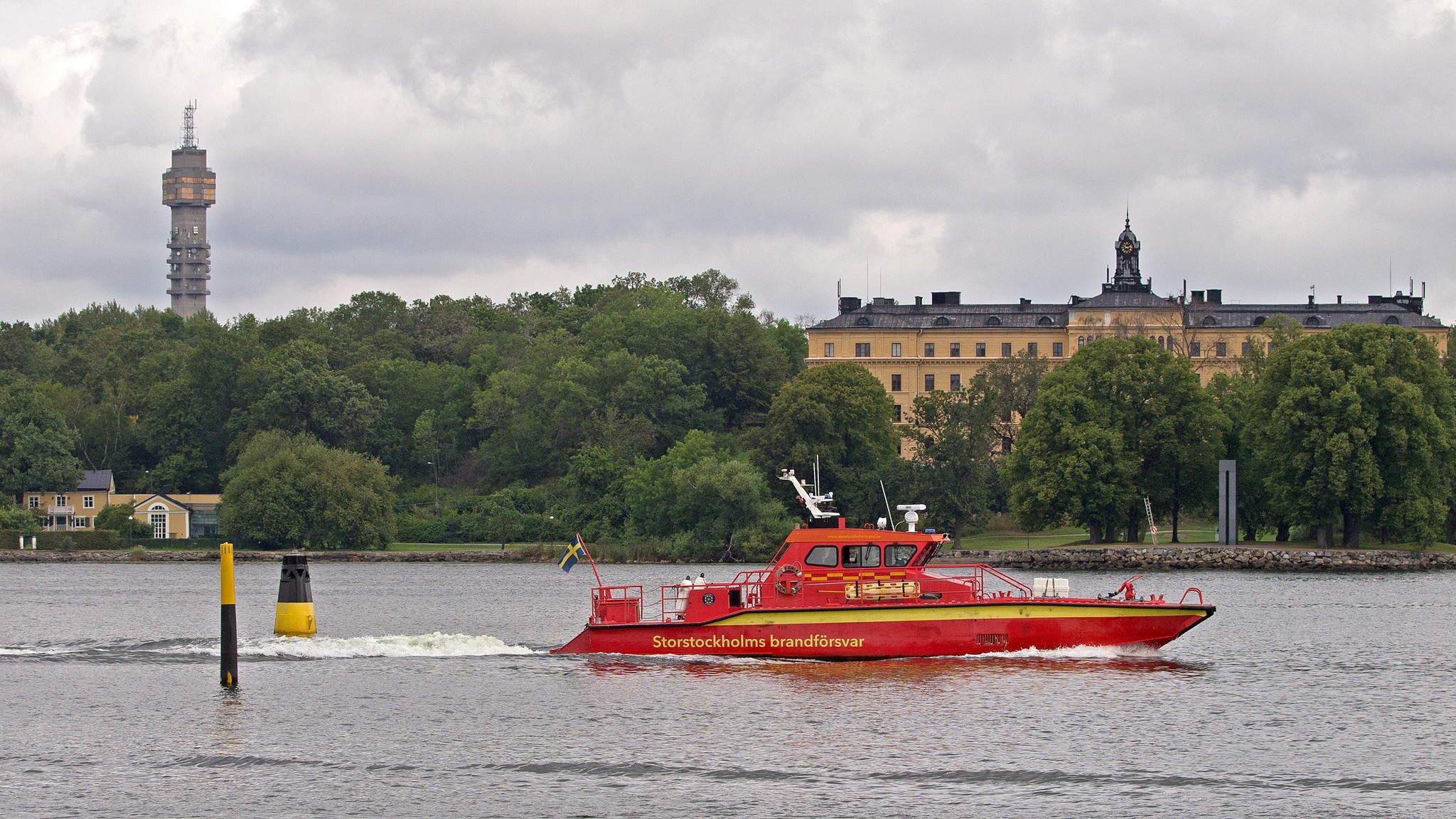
[548,454,1218,663]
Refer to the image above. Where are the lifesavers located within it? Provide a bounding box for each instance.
[775,565,802,594]
